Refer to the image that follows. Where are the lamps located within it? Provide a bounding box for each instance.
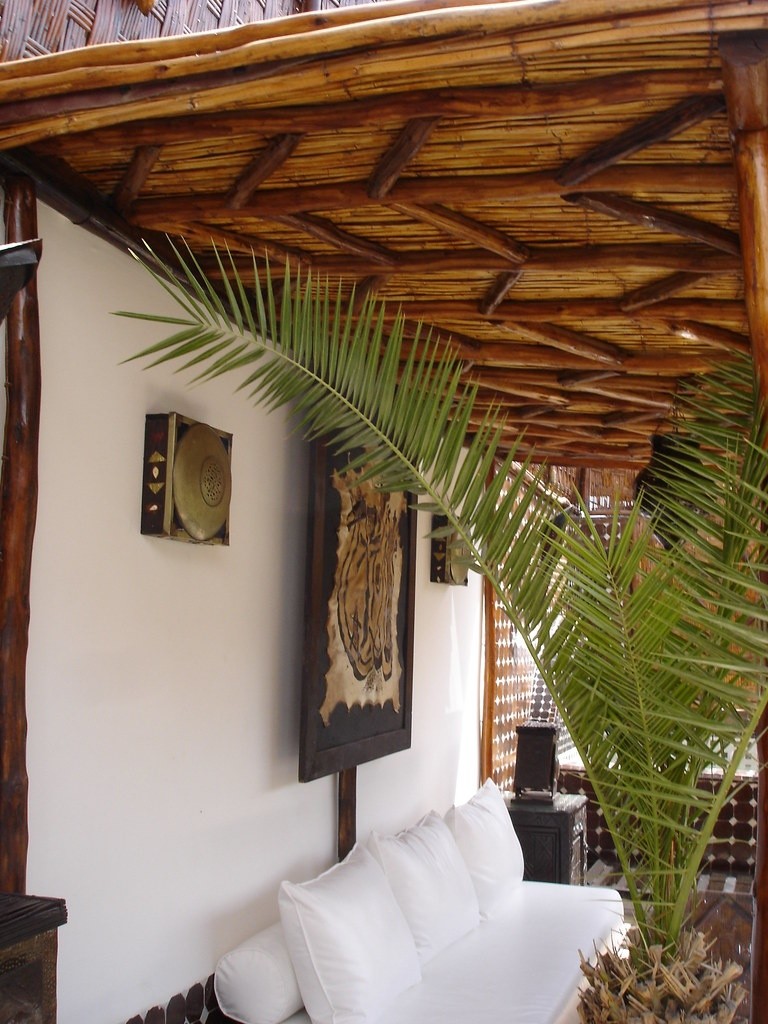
[632,394,715,552]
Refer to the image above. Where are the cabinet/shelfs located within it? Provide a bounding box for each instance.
[1,891,66,1024]
[502,791,588,886]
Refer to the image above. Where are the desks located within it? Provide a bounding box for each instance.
[566,770,757,870]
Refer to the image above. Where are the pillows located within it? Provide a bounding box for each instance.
[366,811,479,965]
[443,778,524,895]
[280,843,420,1024]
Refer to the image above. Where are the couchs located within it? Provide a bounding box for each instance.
[214,882,629,1024]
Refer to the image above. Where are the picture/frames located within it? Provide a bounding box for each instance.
[299,426,419,782]
